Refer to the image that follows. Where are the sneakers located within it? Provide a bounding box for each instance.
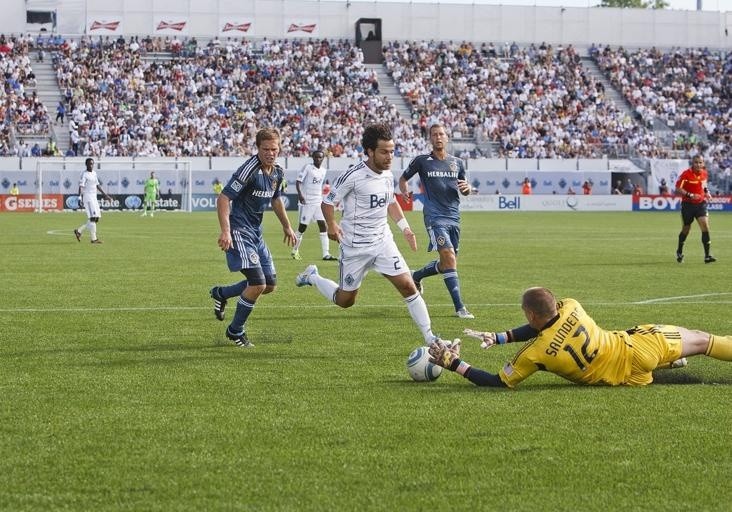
[91,239,104,243]
[677,254,683,262]
[670,356,688,368]
[456,306,474,319]
[140,213,147,216]
[704,256,715,263]
[226,324,255,347]
[323,255,337,260]
[409,270,423,295]
[151,214,153,217]
[210,286,227,320]
[296,264,319,286]
[427,336,452,349]
[74,229,82,242]
[291,251,302,261]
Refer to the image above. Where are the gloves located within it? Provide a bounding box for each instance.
[427,337,462,371]
[462,329,503,349]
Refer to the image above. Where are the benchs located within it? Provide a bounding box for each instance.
[450,132,500,156]
[140,50,175,55]
[141,57,185,65]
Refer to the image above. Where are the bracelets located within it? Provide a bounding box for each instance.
[397,217,410,230]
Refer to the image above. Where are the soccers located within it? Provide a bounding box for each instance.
[407,347,442,381]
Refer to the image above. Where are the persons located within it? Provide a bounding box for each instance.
[140,171,161,218]
[0,28,732,198]
[290,151,337,260]
[210,128,299,348]
[73,158,113,244]
[429,287,732,388]
[296,124,452,348]
[676,154,716,264]
[399,124,474,319]
